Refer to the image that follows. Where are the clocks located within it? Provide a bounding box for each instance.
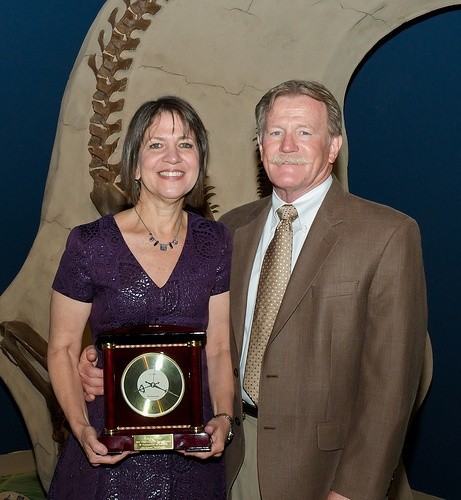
[95,322,211,454]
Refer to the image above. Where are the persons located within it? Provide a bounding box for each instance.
[47,97,234,500]
[77,79,429,500]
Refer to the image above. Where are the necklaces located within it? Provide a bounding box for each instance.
[133,205,182,251]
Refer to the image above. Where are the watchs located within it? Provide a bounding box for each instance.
[213,413,234,443]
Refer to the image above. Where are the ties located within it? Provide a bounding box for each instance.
[242,204,299,407]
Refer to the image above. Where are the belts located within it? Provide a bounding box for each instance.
[242,398,259,420]
